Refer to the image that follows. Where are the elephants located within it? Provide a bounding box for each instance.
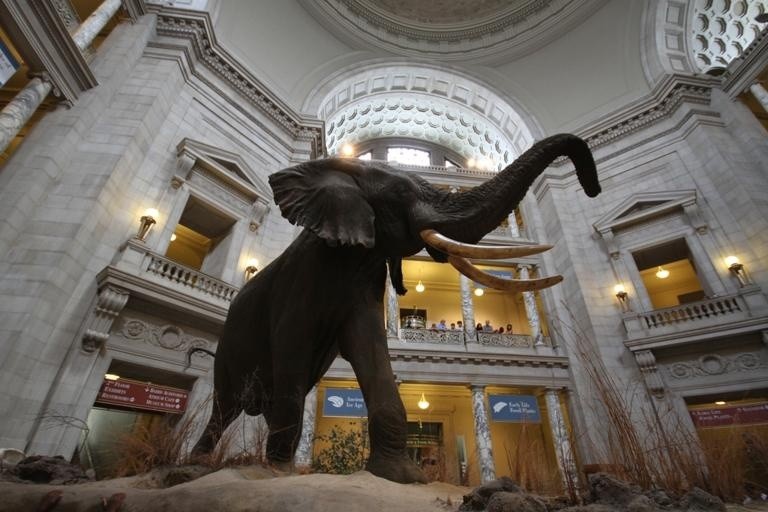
[187,133,603,485]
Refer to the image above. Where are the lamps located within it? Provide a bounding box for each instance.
[417,392,430,410]
[244,257,259,284]
[613,283,634,313]
[104,373,120,381]
[726,255,754,288]
[655,266,670,279]
[415,280,425,293]
[132,208,160,243]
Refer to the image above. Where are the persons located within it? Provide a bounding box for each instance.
[404,319,513,335]
